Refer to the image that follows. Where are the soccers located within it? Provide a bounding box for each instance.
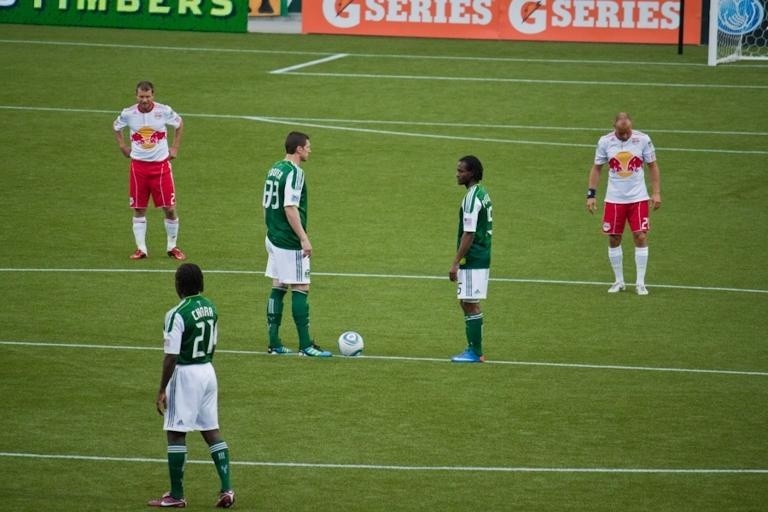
[337,330,364,355]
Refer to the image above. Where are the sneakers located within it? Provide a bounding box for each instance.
[267,345,293,354]
[130,250,146,259]
[148,493,186,507]
[299,344,331,357]
[450,349,484,362]
[636,284,648,295]
[607,282,625,292]
[215,491,235,508]
[167,247,185,260]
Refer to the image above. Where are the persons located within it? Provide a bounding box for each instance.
[147,262,234,507]
[263,132,333,357]
[113,81,186,260]
[587,111,662,294]
[449,155,493,362]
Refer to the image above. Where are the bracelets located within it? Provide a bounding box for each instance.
[587,188,595,198]
